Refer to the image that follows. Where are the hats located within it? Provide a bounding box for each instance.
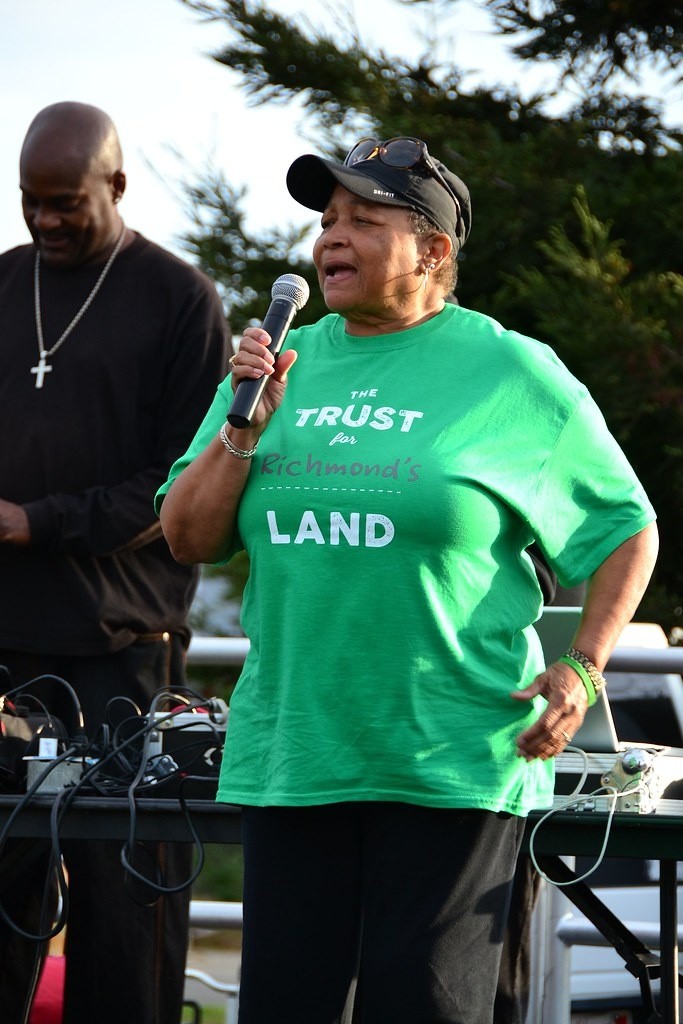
[286,148,472,258]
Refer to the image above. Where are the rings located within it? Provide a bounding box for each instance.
[562,731,569,740]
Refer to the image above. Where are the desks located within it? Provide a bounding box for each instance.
[0,791,683,1024]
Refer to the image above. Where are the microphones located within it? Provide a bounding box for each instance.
[226,274,310,429]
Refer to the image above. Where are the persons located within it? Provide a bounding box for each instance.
[0,99,234,1024]
[154,145,658,1024]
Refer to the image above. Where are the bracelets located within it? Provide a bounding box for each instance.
[220,422,259,459]
[560,647,607,707]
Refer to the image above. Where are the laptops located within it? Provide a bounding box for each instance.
[532,606,672,752]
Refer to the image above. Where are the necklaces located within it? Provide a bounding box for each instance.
[31,221,126,386]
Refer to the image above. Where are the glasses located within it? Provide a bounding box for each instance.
[343,137,463,222]
